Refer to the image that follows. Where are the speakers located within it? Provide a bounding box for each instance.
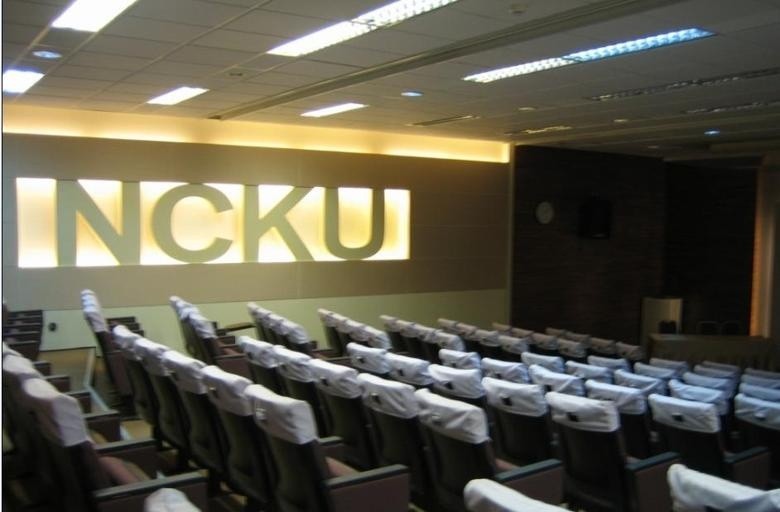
[582,198,610,240]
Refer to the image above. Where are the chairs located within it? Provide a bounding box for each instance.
[3,289,779,510]
